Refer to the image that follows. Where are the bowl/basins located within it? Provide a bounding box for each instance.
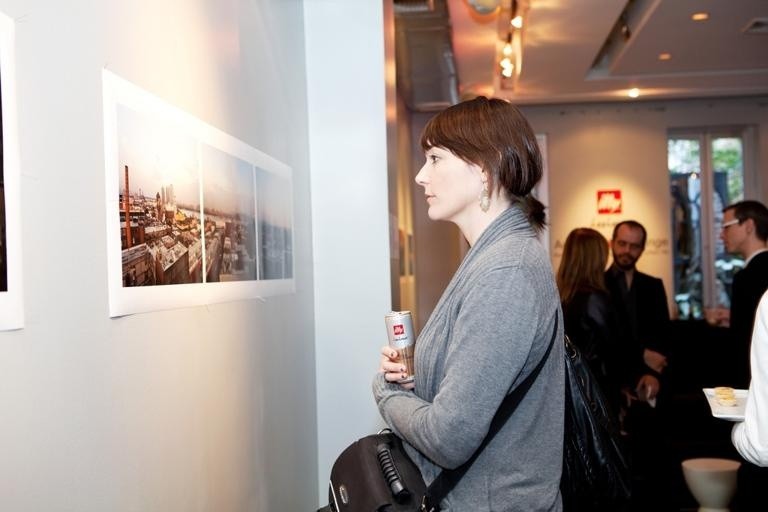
[681,457,740,510]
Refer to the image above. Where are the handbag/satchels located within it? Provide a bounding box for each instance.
[318,427,445,511]
[558,334,634,512]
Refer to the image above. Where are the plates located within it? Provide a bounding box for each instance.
[701,388,750,423]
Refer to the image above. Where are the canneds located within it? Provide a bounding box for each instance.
[384,311,416,384]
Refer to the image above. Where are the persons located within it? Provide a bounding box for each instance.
[374,98,567,511]
